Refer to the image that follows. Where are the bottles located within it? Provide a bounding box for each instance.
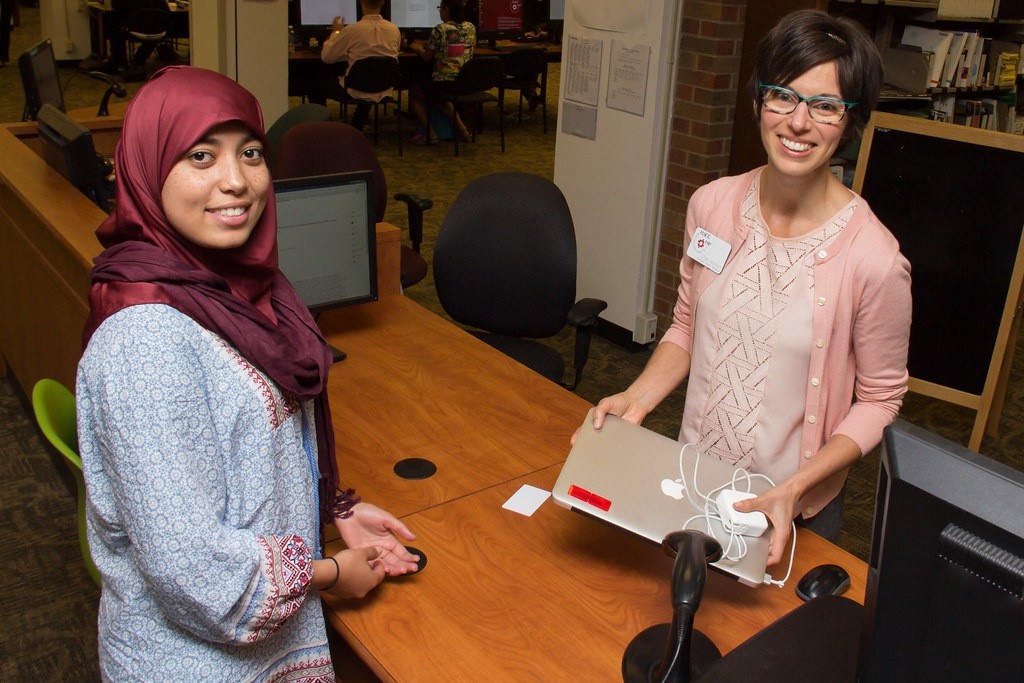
[288,26,295,55]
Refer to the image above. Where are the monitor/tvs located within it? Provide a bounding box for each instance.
[271,170,379,362]
[35,103,110,215]
[18,37,66,121]
[297,0,359,45]
[390,0,444,42]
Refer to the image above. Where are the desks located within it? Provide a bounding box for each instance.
[0,116,872,683]
[83,2,190,79]
[288,42,562,71]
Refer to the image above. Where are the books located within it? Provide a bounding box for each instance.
[902,24,1016,134]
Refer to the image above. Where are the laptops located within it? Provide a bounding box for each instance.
[552,407,773,589]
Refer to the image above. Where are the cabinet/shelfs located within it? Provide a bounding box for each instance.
[824,0,1024,173]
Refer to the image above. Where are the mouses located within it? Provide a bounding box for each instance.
[796,564,851,602]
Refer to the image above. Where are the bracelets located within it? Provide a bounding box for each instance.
[335,31,340,34]
[318,557,339,592]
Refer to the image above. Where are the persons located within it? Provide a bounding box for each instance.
[409,0,476,145]
[109,0,171,68]
[76,65,421,683]
[570,8,912,567]
[307,0,402,131]
[0,0,20,68]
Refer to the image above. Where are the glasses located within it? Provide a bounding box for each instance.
[758,79,858,126]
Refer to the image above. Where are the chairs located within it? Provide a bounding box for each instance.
[263,121,430,288]
[32,378,100,593]
[265,103,330,170]
[107,0,176,84]
[433,172,610,391]
[288,47,549,158]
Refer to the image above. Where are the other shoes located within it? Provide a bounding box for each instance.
[458,130,473,142]
[427,134,440,145]
